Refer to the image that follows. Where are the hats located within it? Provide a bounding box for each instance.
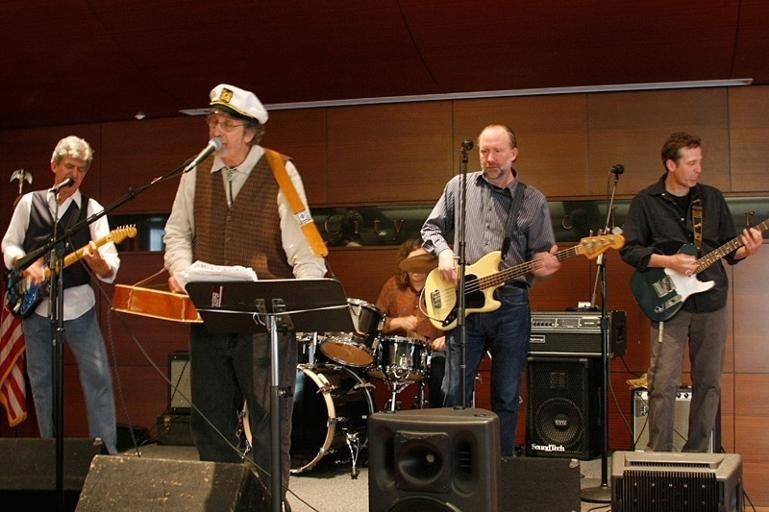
[204,83,268,125]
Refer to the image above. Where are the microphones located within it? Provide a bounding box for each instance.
[180,135,223,176]
[457,136,476,165]
[47,176,74,196]
[607,160,626,180]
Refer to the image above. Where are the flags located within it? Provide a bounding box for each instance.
[0,288,29,428]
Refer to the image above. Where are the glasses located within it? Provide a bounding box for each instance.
[205,115,245,131]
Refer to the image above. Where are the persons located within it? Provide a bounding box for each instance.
[616,131,763,454]
[159,83,330,511]
[0,135,124,460]
[371,237,455,354]
[419,124,563,462]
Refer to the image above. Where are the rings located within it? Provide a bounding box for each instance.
[684,268,691,276]
[451,265,457,270]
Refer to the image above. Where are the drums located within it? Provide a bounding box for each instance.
[381,335,431,380]
[318,339,375,398]
[322,295,384,369]
[288,362,376,475]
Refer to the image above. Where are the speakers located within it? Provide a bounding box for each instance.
[498,455,582,512]
[612,451,744,512]
[73,453,271,512]
[368,406,503,512]
[524,355,609,462]
[0,434,110,494]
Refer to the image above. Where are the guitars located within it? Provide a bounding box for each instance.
[3,224,139,317]
[109,282,202,325]
[629,219,765,325]
[424,227,624,331]
